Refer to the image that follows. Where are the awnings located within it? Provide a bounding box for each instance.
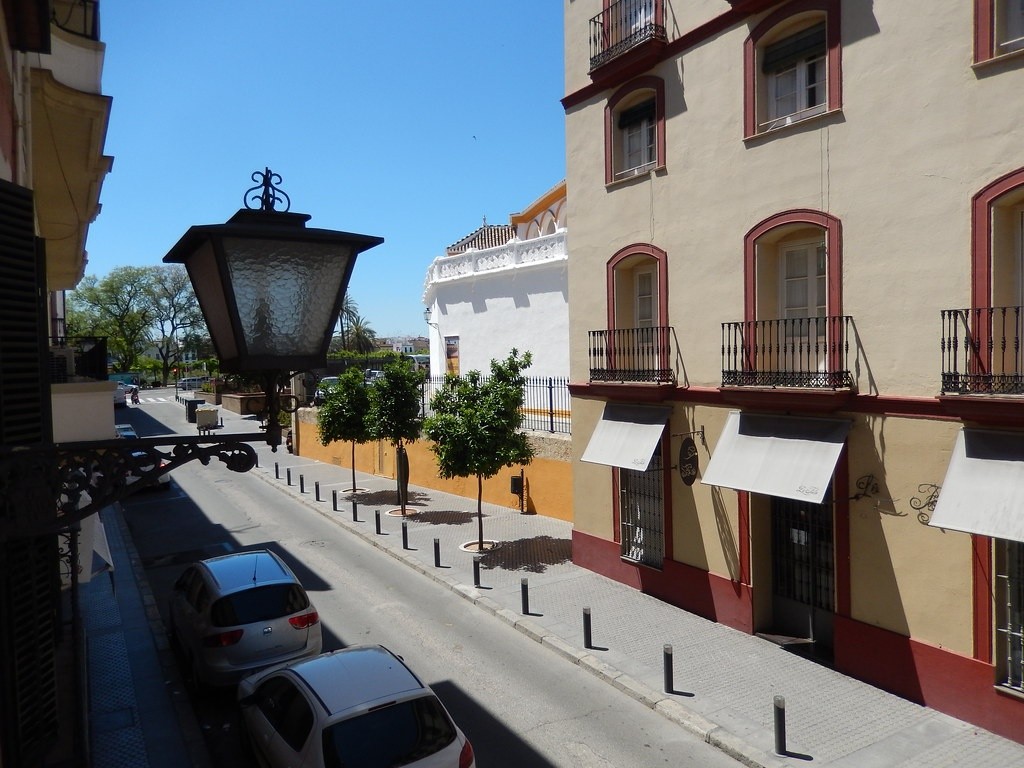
[58,467,114,589]
[700,410,851,505]
[928,426,1024,543]
[579,400,672,474]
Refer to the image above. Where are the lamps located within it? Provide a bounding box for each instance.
[424,308,432,325]
[1,167,382,546]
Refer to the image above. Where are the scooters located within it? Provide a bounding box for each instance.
[129,390,139,405]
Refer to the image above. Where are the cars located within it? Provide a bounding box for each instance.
[178,376,235,392]
[358,369,386,388]
[314,376,346,406]
[118,381,140,394]
[167,549,323,703]
[116,422,171,495]
[238,642,476,768]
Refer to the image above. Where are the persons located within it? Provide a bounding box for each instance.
[131,388,141,404]
